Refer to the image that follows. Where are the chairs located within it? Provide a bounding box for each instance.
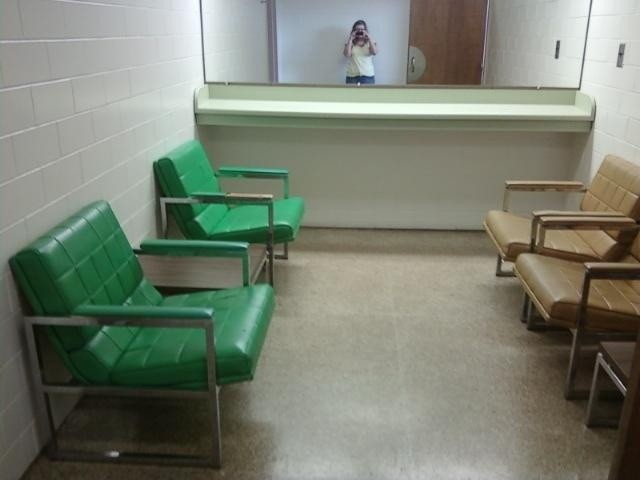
[9,198,273,470]
[509,214,639,400]
[487,155,640,322]
[153,139,305,288]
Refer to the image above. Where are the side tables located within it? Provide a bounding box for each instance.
[135,244,270,289]
[585,340,637,426]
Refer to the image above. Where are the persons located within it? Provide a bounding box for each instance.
[343,20,378,84]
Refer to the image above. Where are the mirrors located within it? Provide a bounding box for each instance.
[200,0,593,92]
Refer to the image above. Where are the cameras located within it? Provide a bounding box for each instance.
[356,31,362,34]
